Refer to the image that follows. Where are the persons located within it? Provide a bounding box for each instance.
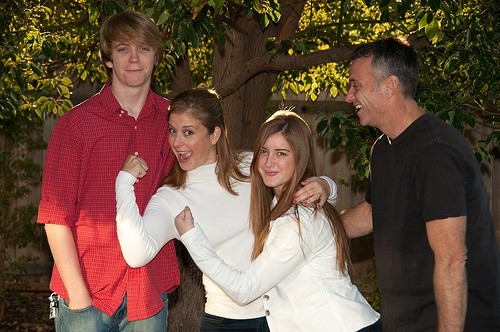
[338,37,499,332]
[170,108,387,332]
[35,9,185,332]
[115,88,354,332]
